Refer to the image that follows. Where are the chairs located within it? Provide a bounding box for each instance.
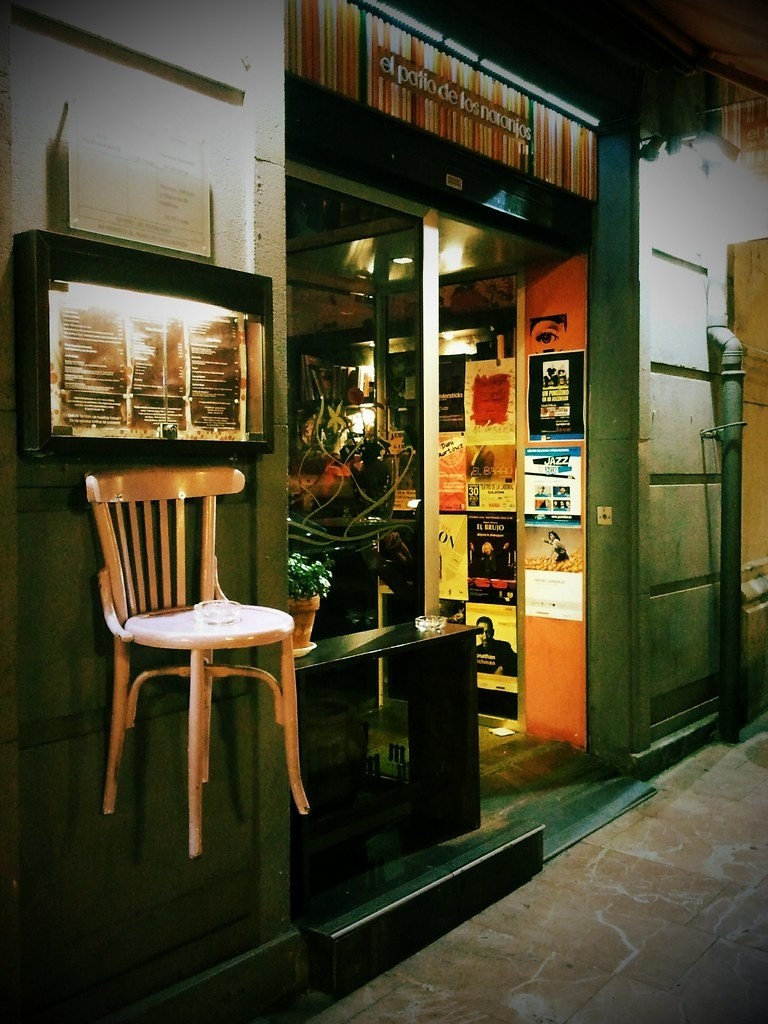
[81,471,320,862]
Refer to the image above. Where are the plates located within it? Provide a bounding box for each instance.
[292,642,317,657]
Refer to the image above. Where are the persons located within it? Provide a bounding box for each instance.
[553,500,571,511]
[476,617,518,677]
[442,604,466,623]
[556,487,569,497]
[535,486,548,497]
[468,542,514,582]
[546,364,566,385]
[542,531,569,563]
[468,445,494,485]
[384,532,412,565]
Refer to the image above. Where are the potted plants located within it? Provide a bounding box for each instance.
[285,542,329,656]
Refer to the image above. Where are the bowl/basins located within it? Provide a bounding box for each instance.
[415,615,448,632]
[195,600,242,626]
